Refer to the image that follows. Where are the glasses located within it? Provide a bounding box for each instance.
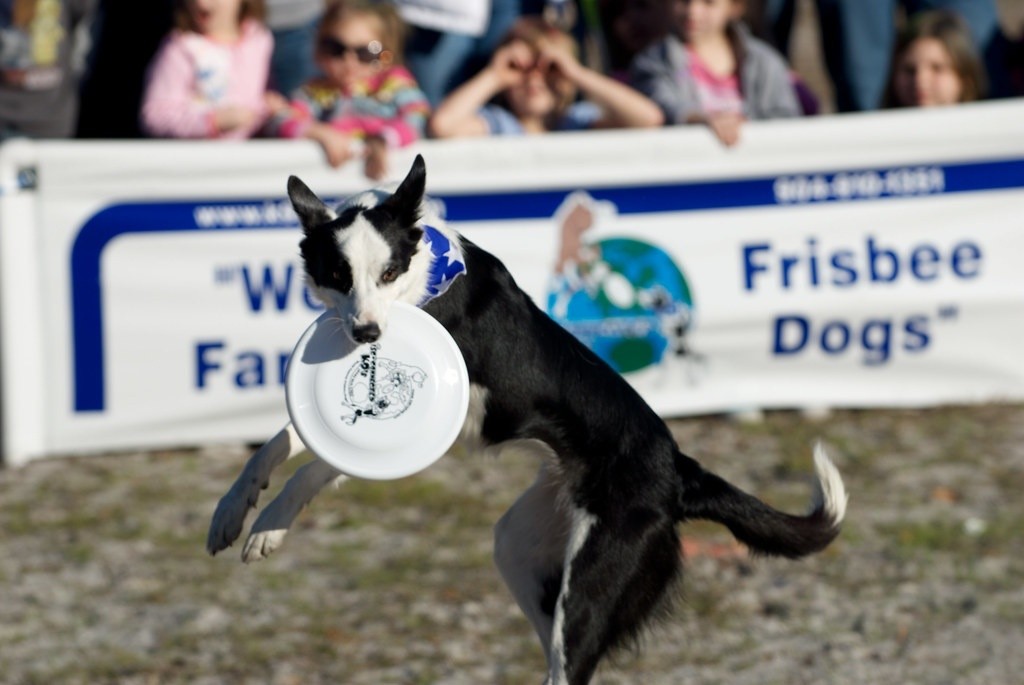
[321,36,390,63]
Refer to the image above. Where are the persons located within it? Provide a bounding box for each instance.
[429,0,668,138]
[142,1,287,140]
[273,0,430,178]
[861,0,1024,108]
[637,0,802,146]
[0,0,93,139]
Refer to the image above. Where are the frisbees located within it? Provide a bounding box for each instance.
[285,302,471,482]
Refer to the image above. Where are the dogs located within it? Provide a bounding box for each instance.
[204,153,847,685]
